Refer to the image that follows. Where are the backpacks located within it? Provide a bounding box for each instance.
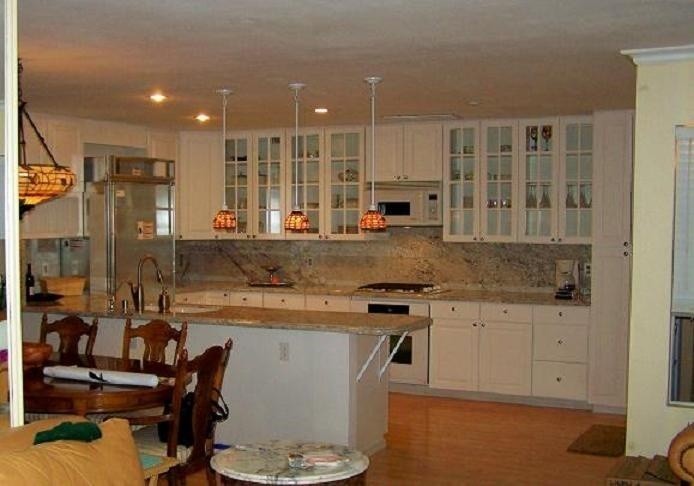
[157,385,228,448]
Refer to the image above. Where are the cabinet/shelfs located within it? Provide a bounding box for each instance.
[181,279,352,312]
[445,116,593,244]
[430,289,589,410]
[365,123,442,227]
[176,115,364,244]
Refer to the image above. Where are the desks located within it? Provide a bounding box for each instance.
[7,353,192,415]
[209,439,370,486]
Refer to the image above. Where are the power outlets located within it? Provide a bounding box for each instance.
[585,263,591,276]
[35,282,42,294]
[279,342,289,361]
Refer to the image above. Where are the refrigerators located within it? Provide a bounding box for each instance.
[88,180,175,295]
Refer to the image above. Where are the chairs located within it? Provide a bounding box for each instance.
[86,316,187,430]
[132,338,233,485]
[24,311,99,421]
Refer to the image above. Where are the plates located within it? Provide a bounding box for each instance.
[245,281,296,287]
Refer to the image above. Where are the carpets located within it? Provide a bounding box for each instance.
[566,424,625,457]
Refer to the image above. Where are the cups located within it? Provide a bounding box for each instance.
[260,265,282,283]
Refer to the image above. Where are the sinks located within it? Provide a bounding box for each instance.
[140,304,225,314]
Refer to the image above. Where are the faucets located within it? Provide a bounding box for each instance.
[136,255,165,315]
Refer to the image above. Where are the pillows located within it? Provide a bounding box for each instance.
[0,418,86,455]
[1,418,145,485]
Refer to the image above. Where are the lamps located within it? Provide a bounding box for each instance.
[359,77,388,230]
[17,57,77,221]
[213,89,237,230]
[284,84,310,231]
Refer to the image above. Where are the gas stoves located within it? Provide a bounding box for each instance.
[353,283,456,298]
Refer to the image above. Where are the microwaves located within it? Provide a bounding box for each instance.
[362,188,443,227]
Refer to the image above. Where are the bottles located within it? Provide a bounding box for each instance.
[107,295,115,314]
[26,263,35,301]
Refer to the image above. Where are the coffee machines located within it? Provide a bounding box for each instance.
[553,259,580,300]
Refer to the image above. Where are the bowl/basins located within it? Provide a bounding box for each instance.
[22,342,52,369]
